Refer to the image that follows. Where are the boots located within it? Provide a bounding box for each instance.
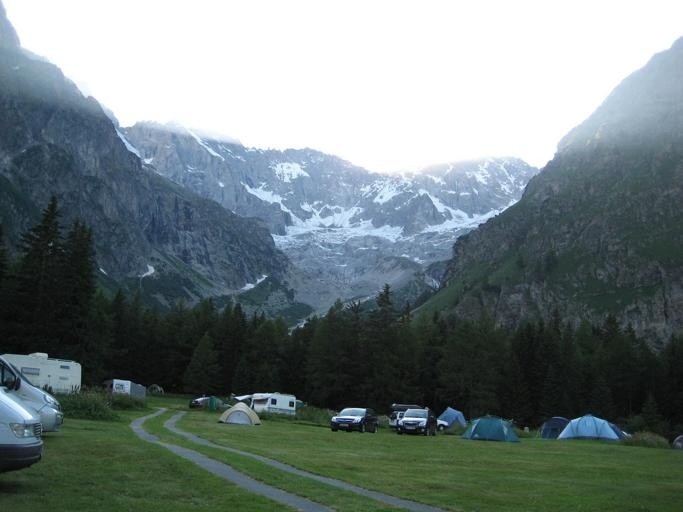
[388,403,449,431]
[397,406,438,437]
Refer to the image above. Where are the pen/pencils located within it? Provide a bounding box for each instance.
[0,350,64,437]
[0,379,45,474]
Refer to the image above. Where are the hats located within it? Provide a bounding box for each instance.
[330,407,380,433]
[190,396,231,410]
